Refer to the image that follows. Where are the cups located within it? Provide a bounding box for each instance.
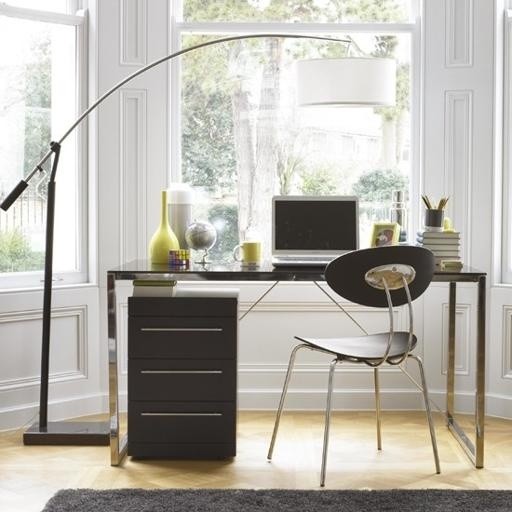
[232,241,262,268]
[424,208,445,233]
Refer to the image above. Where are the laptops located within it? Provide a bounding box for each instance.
[270,196,359,266]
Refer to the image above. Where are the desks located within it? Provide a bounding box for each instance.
[106,256,487,469]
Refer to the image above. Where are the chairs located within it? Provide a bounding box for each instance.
[266,247,442,487]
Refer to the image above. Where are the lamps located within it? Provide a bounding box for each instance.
[0,35,398,447]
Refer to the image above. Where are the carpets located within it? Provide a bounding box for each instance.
[40,489,512,512]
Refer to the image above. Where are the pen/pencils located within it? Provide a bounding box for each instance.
[421,194,451,210]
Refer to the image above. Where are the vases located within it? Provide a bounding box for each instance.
[148,190,181,265]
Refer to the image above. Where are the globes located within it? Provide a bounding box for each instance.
[184,219,217,264]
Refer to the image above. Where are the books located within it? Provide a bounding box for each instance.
[416,231,461,266]
